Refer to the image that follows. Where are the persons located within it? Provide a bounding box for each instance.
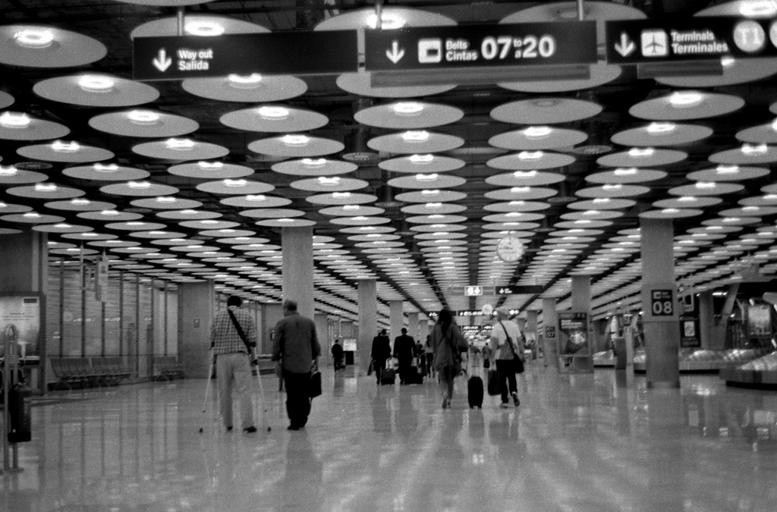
[271,298,322,431]
[207,295,258,433]
[371,306,526,409]
[331,339,345,371]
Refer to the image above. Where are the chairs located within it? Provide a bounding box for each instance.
[51,356,186,390]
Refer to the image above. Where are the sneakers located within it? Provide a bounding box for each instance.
[512,392,519,406]
[441,392,448,408]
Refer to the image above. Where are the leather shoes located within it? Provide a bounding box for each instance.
[288,423,299,430]
[244,427,256,433]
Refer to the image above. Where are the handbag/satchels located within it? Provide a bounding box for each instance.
[487,357,506,395]
[514,352,524,373]
[309,368,321,397]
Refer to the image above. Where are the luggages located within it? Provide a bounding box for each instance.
[467,345,484,409]
[381,358,394,385]
